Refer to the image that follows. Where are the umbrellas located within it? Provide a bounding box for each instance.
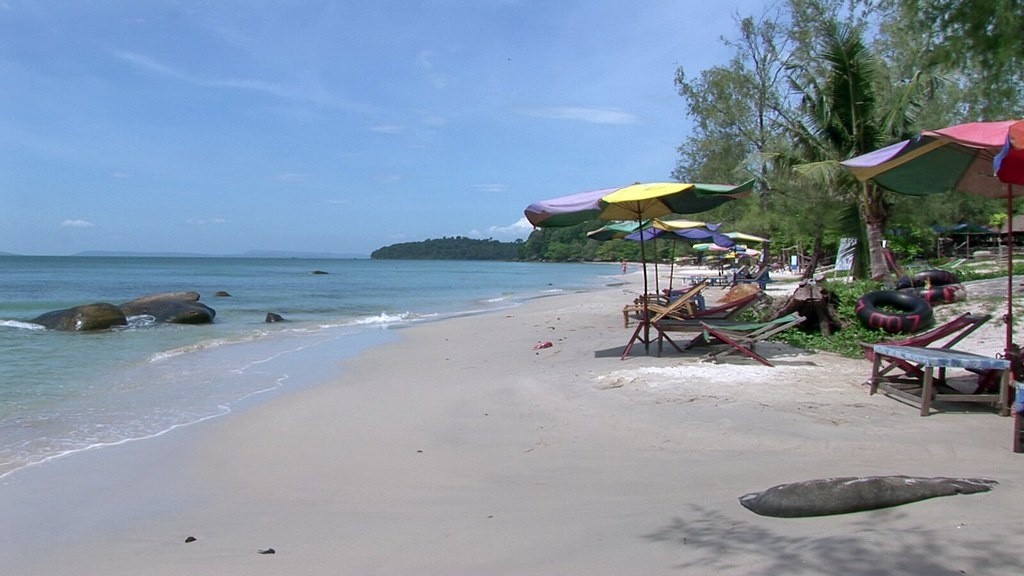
[523,178,757,355]
[720,231,771,253]
[993,119,1024,187]
[943,219,992,261]
[693,243,730,276]
[586,218,724,305]
[673,245,764,275]
[624,227,737,303]
[839,119,1024,360]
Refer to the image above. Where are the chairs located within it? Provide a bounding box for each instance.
[617,262,808,369]
[859,310,993,388]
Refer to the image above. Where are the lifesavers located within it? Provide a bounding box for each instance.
[855,289,932,332]
[892,270,966,304]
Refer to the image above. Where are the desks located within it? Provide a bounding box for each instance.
[869,343,1011,418]
